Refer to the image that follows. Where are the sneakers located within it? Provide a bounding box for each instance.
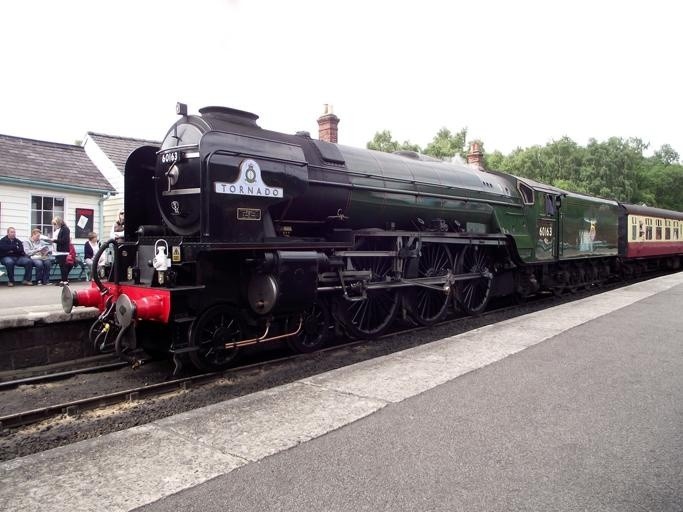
[8,281,70,286]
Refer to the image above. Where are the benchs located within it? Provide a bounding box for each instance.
[0,244,114,282]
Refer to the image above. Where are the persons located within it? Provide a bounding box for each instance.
[0,227,33,287]
[84,232,102,281]
[110,209,124,243]
[51,216,70,285]
[22,228,51,285]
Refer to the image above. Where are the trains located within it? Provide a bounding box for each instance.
[62,102,683,375]
